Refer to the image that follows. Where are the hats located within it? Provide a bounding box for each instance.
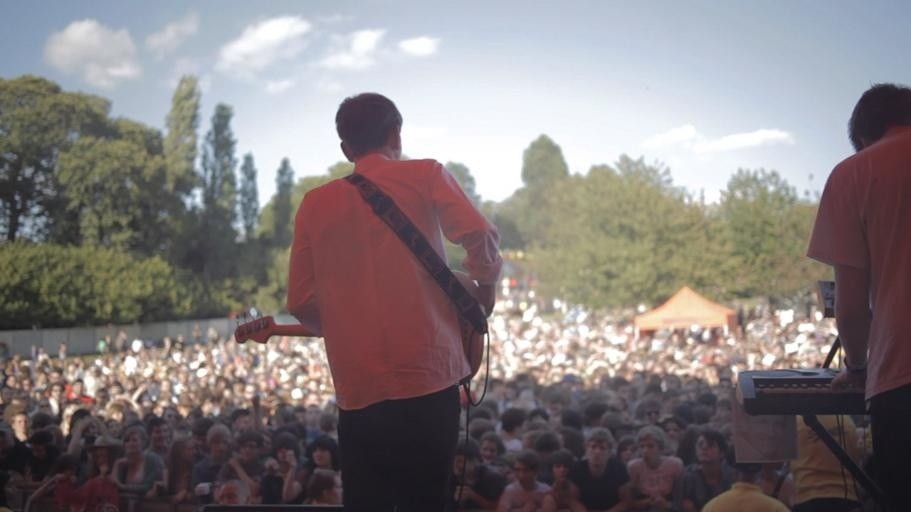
[80,436,124,453]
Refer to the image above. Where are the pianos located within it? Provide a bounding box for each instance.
[739,369,866,414]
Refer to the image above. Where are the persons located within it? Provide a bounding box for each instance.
[283,93,504,510]
[454,289,874,511]
[806,84,910,512]
[0,322,344,511]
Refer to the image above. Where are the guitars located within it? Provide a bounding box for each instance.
[234,270,484,385]
